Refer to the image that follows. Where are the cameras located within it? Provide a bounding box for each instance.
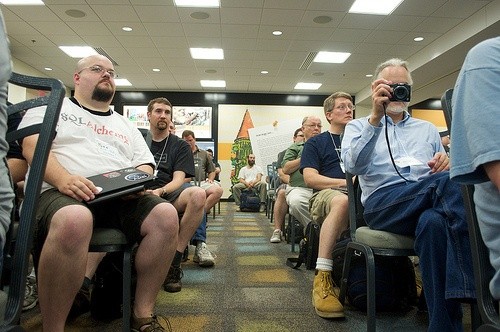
[382,81,411,102]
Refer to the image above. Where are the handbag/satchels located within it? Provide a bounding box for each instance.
[299,220,320,270]
[346,249,416,317]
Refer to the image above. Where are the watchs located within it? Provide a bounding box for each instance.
[160,186,167,198]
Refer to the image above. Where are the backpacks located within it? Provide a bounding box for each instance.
[240,186,260,212]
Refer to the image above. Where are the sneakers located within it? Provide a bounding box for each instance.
[164,265,182,293]
[270,228,281,242]
[193,242,215,266]
[22,276,39,310]
[312,269,345,317]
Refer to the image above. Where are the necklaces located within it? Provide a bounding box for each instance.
[327,129,344,173]
[73,96,118,156]
[151,131,172,181]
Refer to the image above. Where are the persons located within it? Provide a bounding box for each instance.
[17,55,179,332]
[281,116,321,227]
[65,98,207,327]
[168,119,215,266]
[300,92,421,319]
[22,266,38,311]
[342,59,479,332]
[6,107,28,191]
[182,130,223,214]
[270,128,305,242]
[205,149,221,187]
[449,35,500,317]
[0,9,16,264]
[233,154,267,212]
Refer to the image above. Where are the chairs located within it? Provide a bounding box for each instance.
[267,88,500,332]
[0,72,220,332]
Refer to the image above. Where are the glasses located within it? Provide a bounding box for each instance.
[78,65,118,79]
[333,105,356,110]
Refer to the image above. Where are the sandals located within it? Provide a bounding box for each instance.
[131,313,172,332]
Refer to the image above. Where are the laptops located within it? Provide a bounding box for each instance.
[80,166,158,204]
